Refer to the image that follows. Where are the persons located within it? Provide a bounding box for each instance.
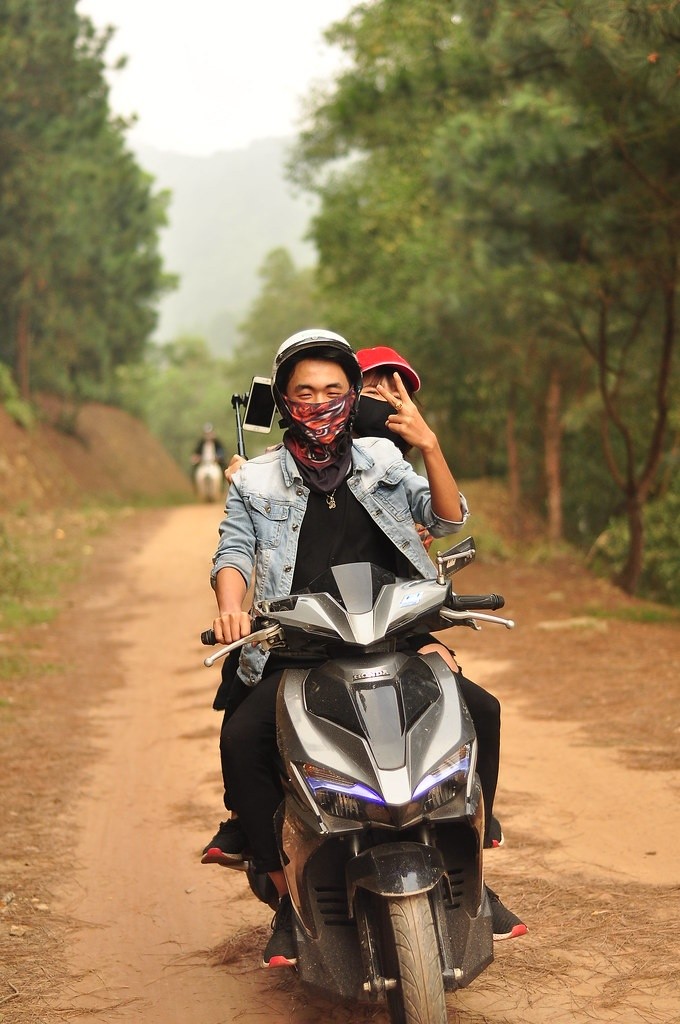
[201,346,504,864]
[192,424,227,482]
[209,327,528,969]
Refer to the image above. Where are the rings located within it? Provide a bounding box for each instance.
[395,403,402,409]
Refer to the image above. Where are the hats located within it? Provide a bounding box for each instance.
[355,346,421,392]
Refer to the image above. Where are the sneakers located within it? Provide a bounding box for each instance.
[483,814,505,849]
[484,884,530,941]
[201,814,248,865]
[261,894,299,968]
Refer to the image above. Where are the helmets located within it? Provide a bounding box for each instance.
[271,329,363,427]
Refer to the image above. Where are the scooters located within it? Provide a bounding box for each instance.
[200,536,515,1024]
[191,454,227,502]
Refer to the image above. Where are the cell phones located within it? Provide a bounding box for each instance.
[242,376,277,434]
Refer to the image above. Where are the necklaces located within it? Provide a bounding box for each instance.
[326,489,336,508]
